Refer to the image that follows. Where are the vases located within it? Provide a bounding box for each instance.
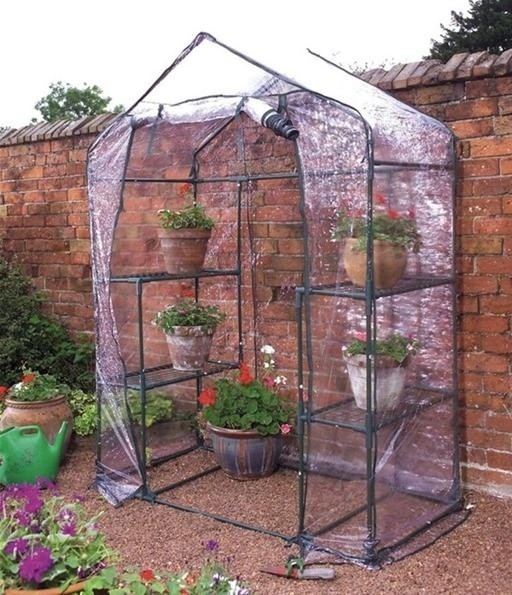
[1,569,88,595]
[343,351,415,413]
[0,396,72,462]
[341,236,409,290]
[162,324,214,371]
[154,227,214,275]
[202,419,286,481]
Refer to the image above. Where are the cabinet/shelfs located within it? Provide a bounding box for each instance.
[98,266,241,396]
[291,273,460,438]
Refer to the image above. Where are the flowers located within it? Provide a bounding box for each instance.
[150,183,213,230]
[155,287,228,338]
[331,185,416,256]
[0,475,115,595]
[0,366,71,402]
[336,318,414,364]
[197,344,294,434]
[79,532,259,594]
